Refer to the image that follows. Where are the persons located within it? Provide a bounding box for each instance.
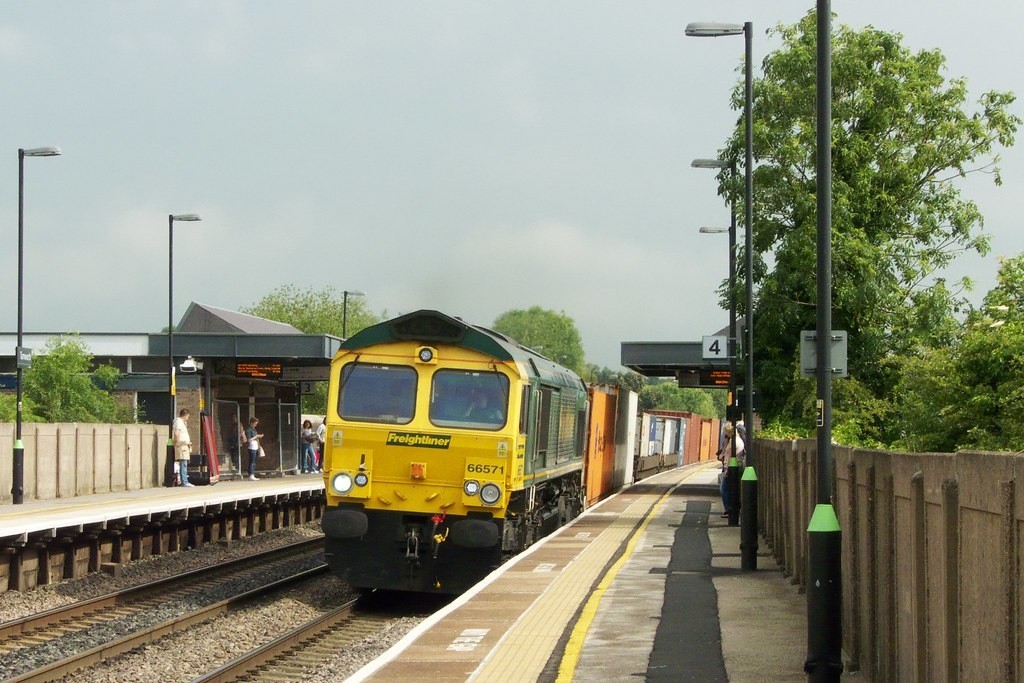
[172,408,197,488]
[718,420,745,518]
[246,417,264,480]
[300,419,320,474]
[316,417,326,473]
[463,390,504,421]
[229,413,247,469]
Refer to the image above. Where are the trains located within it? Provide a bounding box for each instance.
[327,307,729,614]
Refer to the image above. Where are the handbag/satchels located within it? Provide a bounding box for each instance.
[257,447,265,457]
[306,433,319,443]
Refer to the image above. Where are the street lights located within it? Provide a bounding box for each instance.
[164,209,201,450]
[13,145,60,413]
[685,19,758,582]
[340,290,366,338]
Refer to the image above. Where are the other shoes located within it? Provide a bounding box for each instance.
[184,482,195,487]
[319,468,323,472]
[311,470,319,474]
[304,470,309,473]
[248,476,260,481]
[720,511,729,518]
